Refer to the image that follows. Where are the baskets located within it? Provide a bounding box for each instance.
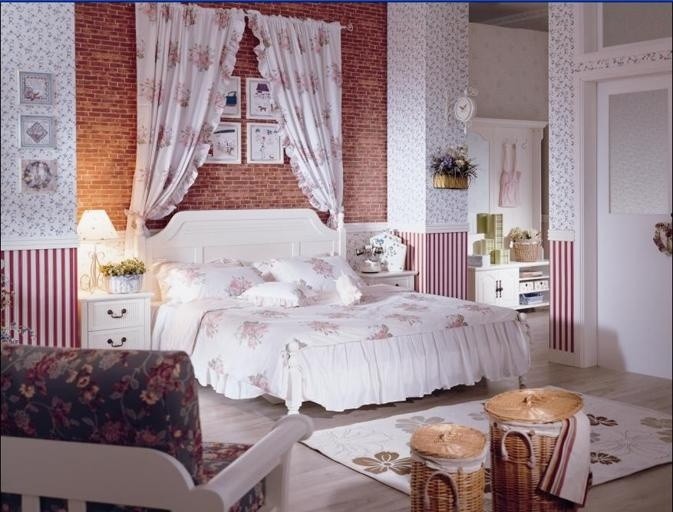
[491,421,579,511]
[410,453,484,511]
[514,241,539,261]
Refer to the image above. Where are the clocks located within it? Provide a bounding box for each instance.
[455,86,478,135]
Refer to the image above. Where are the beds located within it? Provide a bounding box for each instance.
[143,207,532,415]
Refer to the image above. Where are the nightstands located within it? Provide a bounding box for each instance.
[79,292,153,353]
[358,270,419,294]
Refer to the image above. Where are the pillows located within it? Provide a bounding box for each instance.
[148,257,266,296]
[253,253,359,291]
[243,282,298,308]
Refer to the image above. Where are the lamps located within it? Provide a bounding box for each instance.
[77,209,118,291]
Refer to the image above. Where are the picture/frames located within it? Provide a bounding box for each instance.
[246,122,285,165]
[245,77,282,120]
[18,72,53,106]
[221,76,242,119]
[18,158,59,195]
[203,122,242,165]
[19,115,53,147]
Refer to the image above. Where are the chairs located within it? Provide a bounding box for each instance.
[1,340,312,510]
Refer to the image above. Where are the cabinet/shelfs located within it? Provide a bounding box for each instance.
[469,260,518,314]
[517,263,550,307]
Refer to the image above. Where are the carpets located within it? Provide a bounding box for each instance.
[302,387,673,511]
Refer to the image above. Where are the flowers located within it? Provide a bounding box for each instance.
[511,226,543,249]
[435,146,473,174]
[98,257,147,274]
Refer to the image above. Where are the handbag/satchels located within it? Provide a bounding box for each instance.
[499,169,523,207]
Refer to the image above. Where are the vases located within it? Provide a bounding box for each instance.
[515,242,538,262]
[107,275,142,293]
[432,174,469,189]
